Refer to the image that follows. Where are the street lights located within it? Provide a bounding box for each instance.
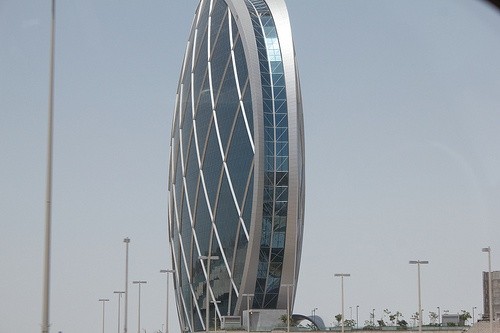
[335,273,351,333]
[314,308,318,322]
[437,306,440,325]
[160,269,176,333]
[198,255,220,332]
[133,280,147,333]
[373,309,375,325]
[357,306,360,330]
[113,290,126,333]
[481,246,494,326]
[473,307,477,325]
[98,298,109,333]
[409,259,429,331]
